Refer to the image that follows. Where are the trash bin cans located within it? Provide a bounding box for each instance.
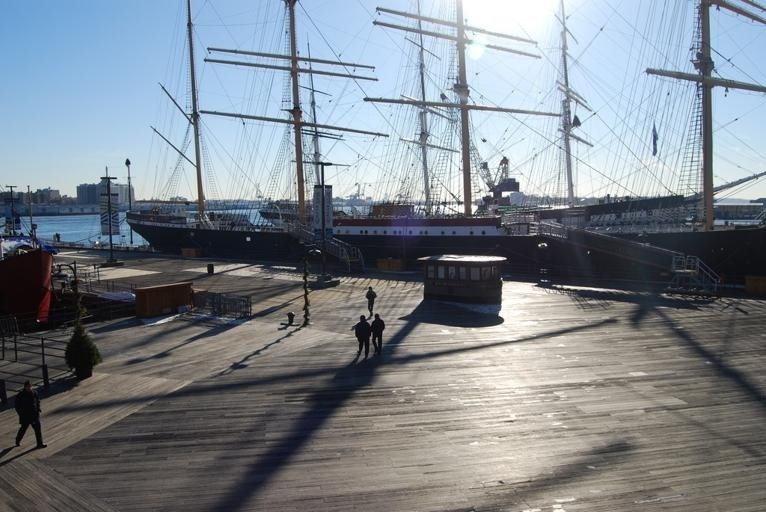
[208,264,213,275]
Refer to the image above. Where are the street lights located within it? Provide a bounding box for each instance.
[125,157,135,245]
[298,242,321,326]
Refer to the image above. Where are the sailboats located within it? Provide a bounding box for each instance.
[126,0,766,281]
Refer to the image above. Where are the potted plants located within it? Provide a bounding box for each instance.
[64,320,103,379]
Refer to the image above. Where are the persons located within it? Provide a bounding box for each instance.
[366,287,377,316]
[369,312,385,355]
[354,314,372,359]
[14,380,47,449]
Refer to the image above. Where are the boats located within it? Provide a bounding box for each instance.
[259,197,352,227]
